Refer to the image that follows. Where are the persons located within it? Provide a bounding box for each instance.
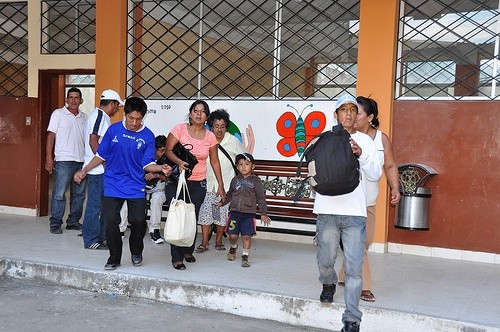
[337,96,402,303]
[72,97,172,270]
[214,152,271,268]
[294,95,401,332]
[44,87,89,235]
[195,108,248,254]
[78,89,125,250]
[146,89,226,271]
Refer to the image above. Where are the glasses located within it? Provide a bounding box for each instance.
[212,124,226,130]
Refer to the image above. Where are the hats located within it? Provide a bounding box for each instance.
[334,94,359,111]
[235,153,255,164]
[100,88,124,107]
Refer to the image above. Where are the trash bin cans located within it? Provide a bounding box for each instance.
[394,185,433,232]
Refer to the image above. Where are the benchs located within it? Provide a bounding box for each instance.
[143,159,322,237]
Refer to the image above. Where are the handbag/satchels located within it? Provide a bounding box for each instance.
[233,165,240,176]
[163,169,196,248]
[156,141,199,186]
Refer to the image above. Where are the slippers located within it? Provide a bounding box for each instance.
[215,244,226,250]
[194,244,208,253]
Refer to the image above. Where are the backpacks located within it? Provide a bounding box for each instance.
[294,122,362,203]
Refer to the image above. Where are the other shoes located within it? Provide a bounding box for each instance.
[172,260,186,270]
[185,255,195,263]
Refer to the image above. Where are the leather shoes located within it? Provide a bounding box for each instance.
[132,253,142,266]
[103,258,121,270]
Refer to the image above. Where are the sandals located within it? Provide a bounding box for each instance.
[360,290,376,302]
[337,281,345,287]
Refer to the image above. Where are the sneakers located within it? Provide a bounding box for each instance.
[150,229,164,244]
[85,239,110,250]
[341,320,360,332]
[227,243,238,261]
[320,283,336,303]
[120,231,124,238]
[50,226,63,235]
[66,222,83,230]
[241,255,250,267]
